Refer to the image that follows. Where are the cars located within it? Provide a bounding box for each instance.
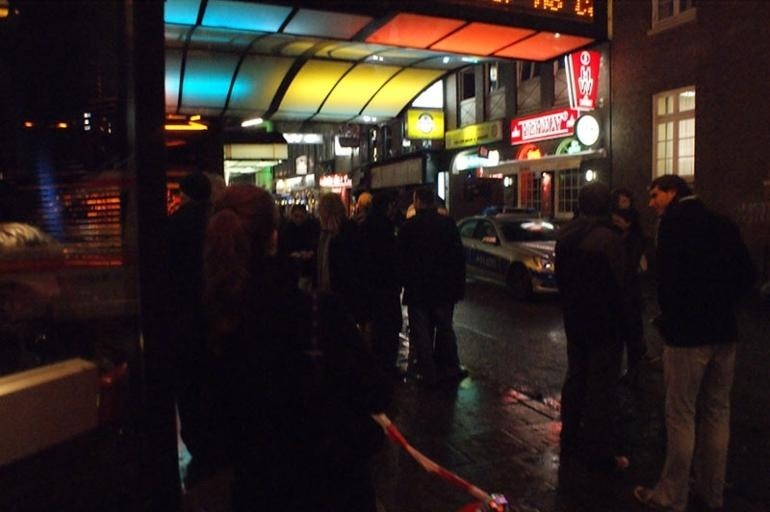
[455,214,563,301]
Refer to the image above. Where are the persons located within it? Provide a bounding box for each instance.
[166,166,470,511]
[1,222,128,511]
[552,183,647,473]
[631,172,755,512]
